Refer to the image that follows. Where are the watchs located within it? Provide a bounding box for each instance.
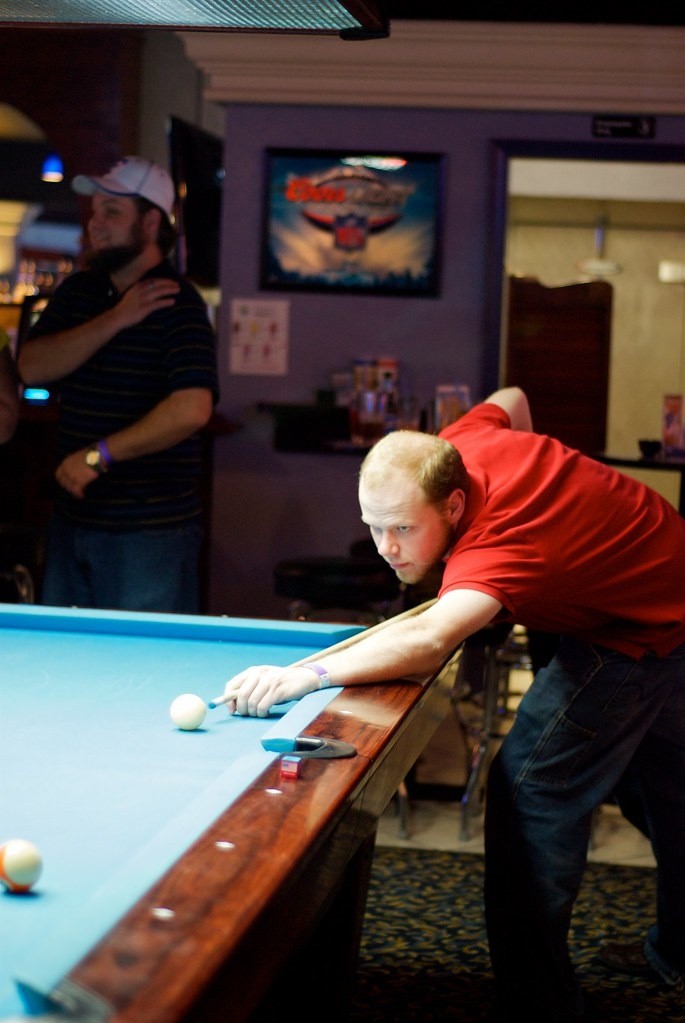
[85,446,107,475]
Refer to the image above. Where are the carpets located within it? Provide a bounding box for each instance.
[342,844,685,1023]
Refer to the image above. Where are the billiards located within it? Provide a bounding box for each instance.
[1,840,43,892]
[171,694,207,730]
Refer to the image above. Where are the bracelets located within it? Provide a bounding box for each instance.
[304,665,330,689]
[100,440,116,466]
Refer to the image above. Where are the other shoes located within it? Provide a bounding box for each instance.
[467,979,576,1023]
[642,925,685,990]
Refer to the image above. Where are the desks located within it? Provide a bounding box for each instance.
[595,451,685,519]
[0,599,466,1023]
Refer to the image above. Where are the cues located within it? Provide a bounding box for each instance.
[208,598,439,708]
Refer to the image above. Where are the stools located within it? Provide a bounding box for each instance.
[459,632,565,838]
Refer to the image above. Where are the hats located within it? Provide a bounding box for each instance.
[72,155,175,215]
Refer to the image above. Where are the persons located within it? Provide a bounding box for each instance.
[225,386,684,1023]
[15,156,221,615]
[0,326,23,445]
